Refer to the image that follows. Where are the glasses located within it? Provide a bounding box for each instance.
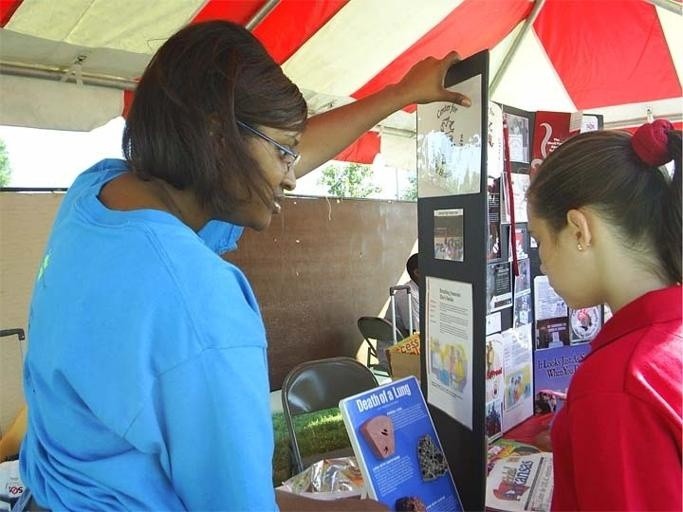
[237,119,302,170]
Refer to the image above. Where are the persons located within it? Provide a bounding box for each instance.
[374,251,419,376]
[518,116,682,511]
[12,15,472,511]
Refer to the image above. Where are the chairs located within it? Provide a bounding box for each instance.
[279,316,407,480]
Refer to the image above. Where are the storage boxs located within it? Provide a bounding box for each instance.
[272,457,391,512]
[386,333,421,384]
